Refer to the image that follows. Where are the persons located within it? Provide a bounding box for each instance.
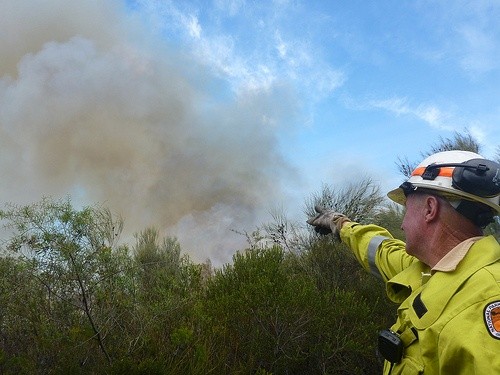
[306,149,500,375]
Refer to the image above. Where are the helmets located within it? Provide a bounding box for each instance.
[387,150,500,214]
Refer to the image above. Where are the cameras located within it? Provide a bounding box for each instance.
[377,330,403,363]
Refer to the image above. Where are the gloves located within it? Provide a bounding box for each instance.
[306,204,350,239]
